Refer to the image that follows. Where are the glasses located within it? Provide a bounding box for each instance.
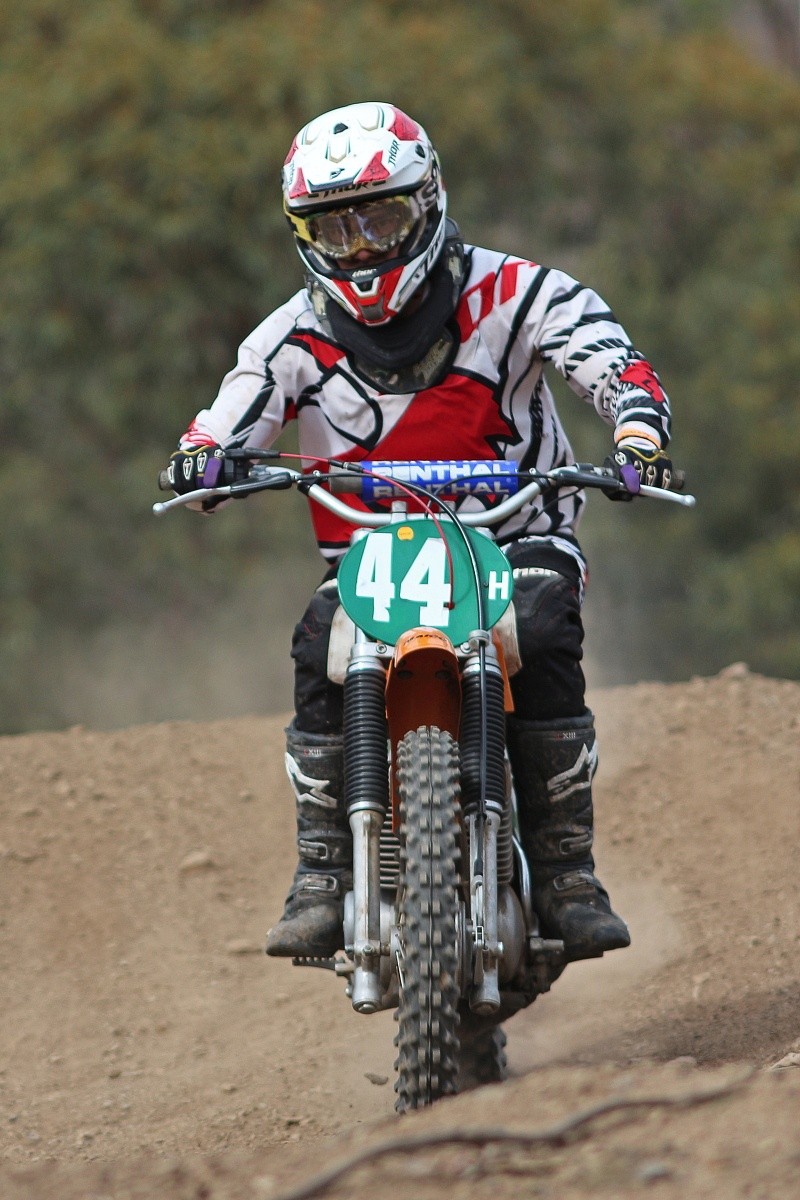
[305,195,421,262]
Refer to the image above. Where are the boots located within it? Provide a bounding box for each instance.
[265,717,354,956]
[506,706,631,950]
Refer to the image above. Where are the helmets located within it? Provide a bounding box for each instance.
[281,101,447,326]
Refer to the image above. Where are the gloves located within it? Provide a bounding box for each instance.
[169,443,236,515]
[601,437,672,501]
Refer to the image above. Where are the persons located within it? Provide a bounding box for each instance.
[166,101,672,957]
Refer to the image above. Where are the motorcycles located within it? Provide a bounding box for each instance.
[147,447,698,1119]
